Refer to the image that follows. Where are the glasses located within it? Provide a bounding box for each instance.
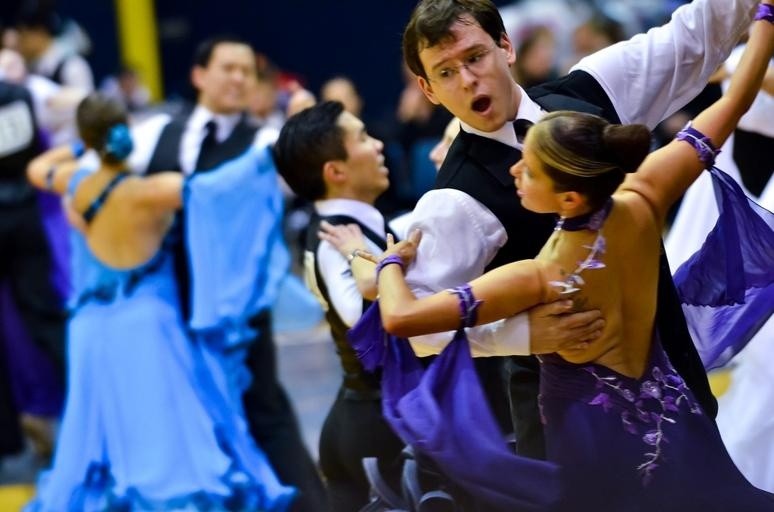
[420,39,500,94]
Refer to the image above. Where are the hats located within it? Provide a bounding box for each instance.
[193,121,222,174]
